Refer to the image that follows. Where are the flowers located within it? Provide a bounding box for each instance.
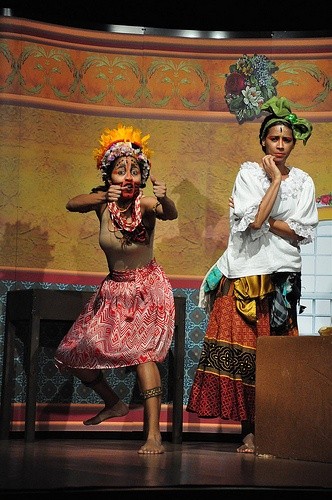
[225,52,279,124]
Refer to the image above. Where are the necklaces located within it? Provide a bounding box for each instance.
[282,166,290,177]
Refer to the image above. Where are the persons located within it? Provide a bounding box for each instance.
[54,127,179,456]
[185,97,318,454]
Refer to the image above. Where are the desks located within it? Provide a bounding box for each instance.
[0,290,187,442]
[254,336,332,464]
